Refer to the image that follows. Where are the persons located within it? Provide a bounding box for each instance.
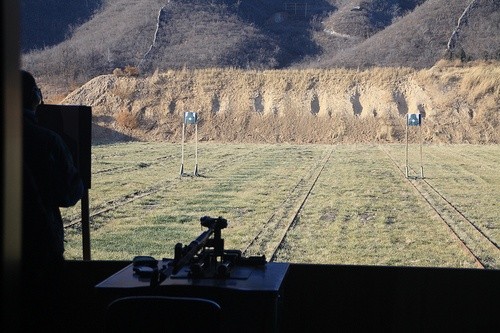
[1,67,83,333]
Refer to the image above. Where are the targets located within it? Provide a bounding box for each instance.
[183,111,197,124]
[406,113,421,126]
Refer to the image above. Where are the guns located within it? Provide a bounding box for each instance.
[172,216,230,281]
